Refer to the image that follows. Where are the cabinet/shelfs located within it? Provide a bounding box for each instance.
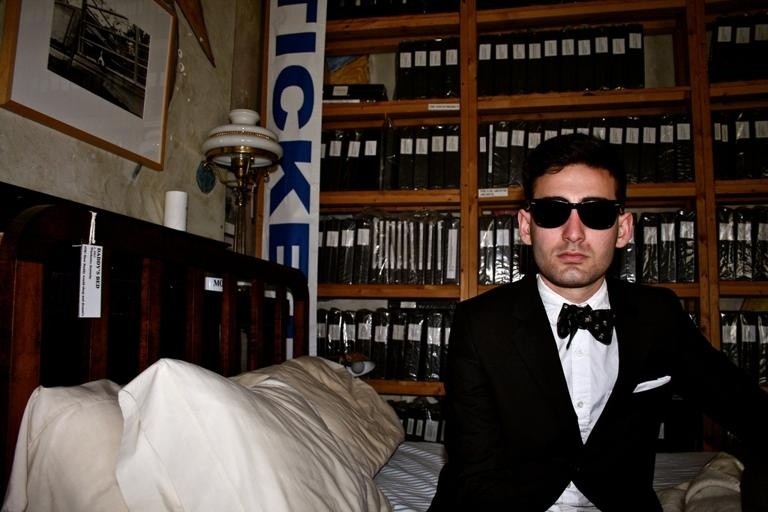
[258,0,467,447]
[467,0,710,344]
[698,0,767,397]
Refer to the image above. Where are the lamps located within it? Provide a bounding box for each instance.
[201,109,282,255]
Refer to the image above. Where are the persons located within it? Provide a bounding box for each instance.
[426,132,768,512]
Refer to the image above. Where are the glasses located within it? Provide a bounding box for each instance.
[524,199,624,229]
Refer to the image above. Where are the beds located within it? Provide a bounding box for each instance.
[0,180,767,510]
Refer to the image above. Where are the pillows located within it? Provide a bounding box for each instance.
[115,356,407,512]
[3,376,129,512]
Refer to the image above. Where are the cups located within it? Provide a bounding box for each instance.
[163,190,188,232]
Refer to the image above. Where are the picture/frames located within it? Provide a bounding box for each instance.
[0,0,178,171]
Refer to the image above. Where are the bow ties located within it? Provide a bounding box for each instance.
[556,302,614,349]
